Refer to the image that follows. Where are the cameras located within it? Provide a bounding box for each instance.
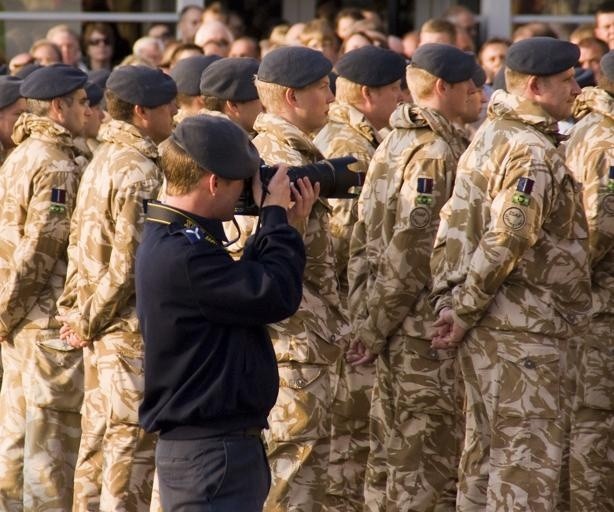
[232,156,368,218]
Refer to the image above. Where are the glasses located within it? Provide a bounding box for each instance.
[89,38,111,45]
[158,32,170,41]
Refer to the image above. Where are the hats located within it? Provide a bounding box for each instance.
[201,58,260,101]
[0,75,21,108]
[600,49,614,82]
[172,114,261,178]
[106,66,177,106]
[87,68,111,87]
[257,47,332,88]
[472,63,487,87]
[15,64,42,78]
[83,82,103,106]
[336,45,407,87]
[19,63,88,98]
[492,64,506,91]
[171,55,224,96]
[506,38,581,76]
[412,43,477,82]
[574,68,593,83]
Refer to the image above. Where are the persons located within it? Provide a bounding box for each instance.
[133,115,319,511]
[0,64,93,512]
[557,48,614,511]
[0,75,27,166]
[54,66,179,512]
[429,36,593,512]
[345,44,475,512]
[72,81,105,174]
[0,4,614,82]
[311,46,406,512]
[155,56,222,156]
[197,57,265,133]
[227,46,336,512]
